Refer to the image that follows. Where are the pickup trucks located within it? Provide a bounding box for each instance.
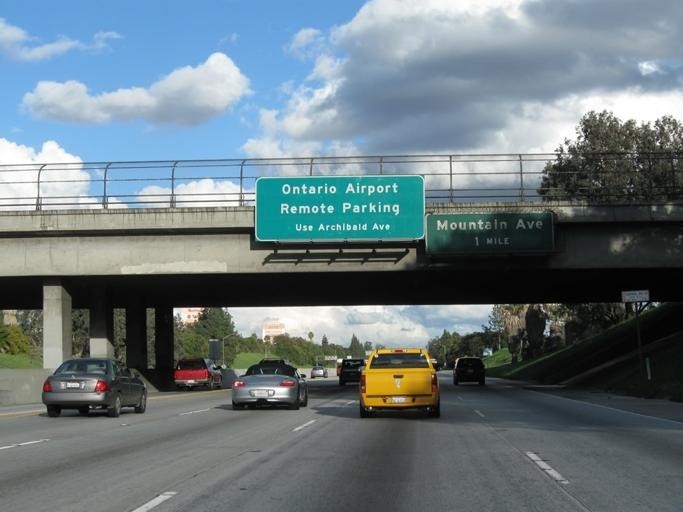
[356,343,444,420]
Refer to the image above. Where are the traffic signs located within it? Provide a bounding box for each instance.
[253,174,424,243]
[423,210,556,257]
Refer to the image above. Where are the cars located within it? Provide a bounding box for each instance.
[39,356,150,418]
[229,359,309,410]
[310,366,328,379]
[336,365,341,376]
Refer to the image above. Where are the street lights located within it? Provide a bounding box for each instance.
[221,333,239,368]
[264,342,277,359]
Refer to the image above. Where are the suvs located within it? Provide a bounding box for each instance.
[338,358,367,387]
[450,355,488,387]
[174,358,224,391]
